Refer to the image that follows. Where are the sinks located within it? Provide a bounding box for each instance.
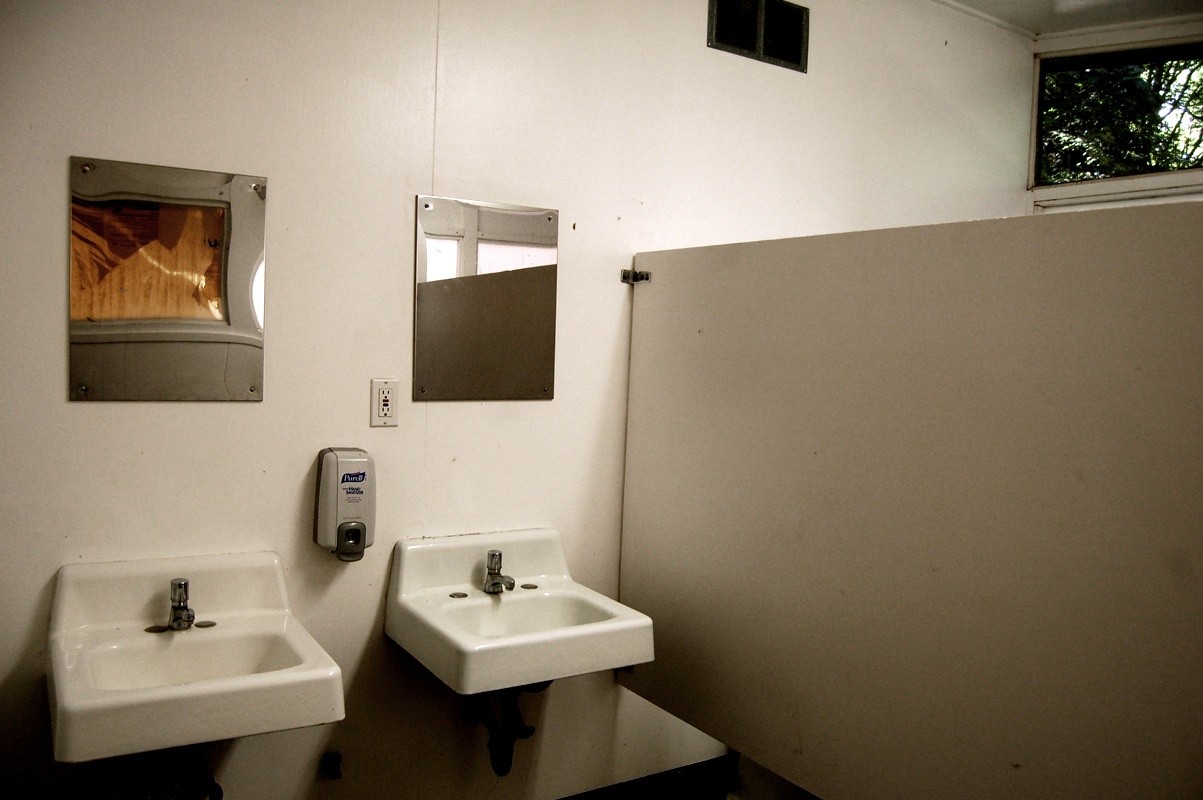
[48,611,346,764]
[383,577,656,696]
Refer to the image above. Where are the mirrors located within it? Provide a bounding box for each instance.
[411,191,560,404]
[63,154,268,406]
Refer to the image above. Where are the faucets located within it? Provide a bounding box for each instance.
[483,548,516,594]
[168,577,196,633]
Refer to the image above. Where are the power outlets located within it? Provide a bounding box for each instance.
[367,377,401,429]
